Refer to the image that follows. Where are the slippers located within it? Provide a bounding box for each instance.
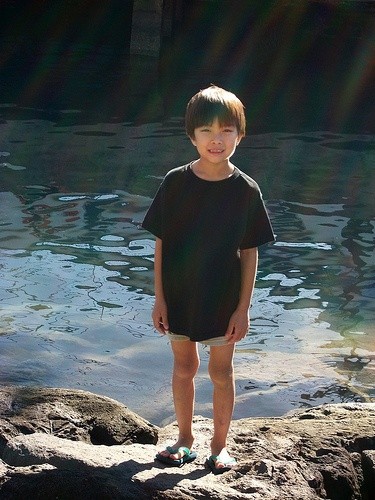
[156,446,197,465]
[206,453,235,473]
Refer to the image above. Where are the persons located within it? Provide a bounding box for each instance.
[141,84,277,475]
